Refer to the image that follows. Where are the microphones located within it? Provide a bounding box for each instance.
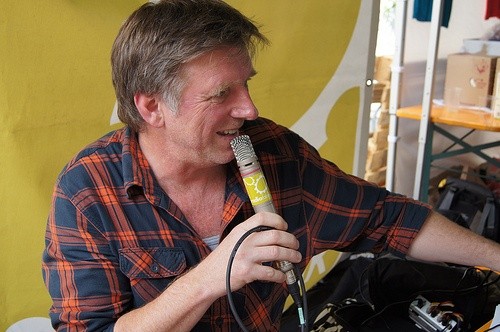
[230,135,302,307]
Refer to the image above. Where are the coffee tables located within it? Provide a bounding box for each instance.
[396,104,500,201]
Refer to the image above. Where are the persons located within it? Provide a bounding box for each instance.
[41,0,499,331]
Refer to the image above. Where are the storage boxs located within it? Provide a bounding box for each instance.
[444,54,500,111]
